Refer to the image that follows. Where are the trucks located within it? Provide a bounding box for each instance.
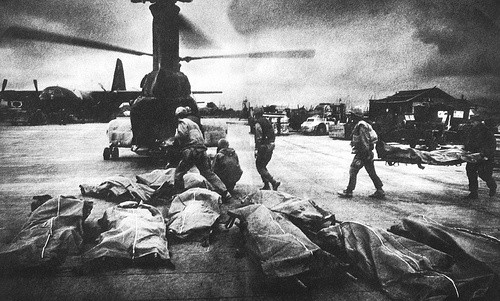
[301,114,348,136]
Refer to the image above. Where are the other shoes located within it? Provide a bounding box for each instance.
[220,191,232,203]
[464,190,479,200]
[272,178,280,191]
[260,181,270,190]
[369,189,386,198]
[487,187,497,198]
[337,189,353,199]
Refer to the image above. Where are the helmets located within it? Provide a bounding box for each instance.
[174,107,189,118]
[349,106,364,119]
[251,106,264,117]
[217,137,229,150]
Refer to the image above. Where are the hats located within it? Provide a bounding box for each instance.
[469,114,482,121]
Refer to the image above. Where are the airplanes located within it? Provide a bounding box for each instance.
[0,58,224,125]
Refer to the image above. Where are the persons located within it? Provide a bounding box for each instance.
[337,106,385,198]
[210,138,242,194]
[253,106,281,192]
[462,114,497,201]
[172,106,232,203]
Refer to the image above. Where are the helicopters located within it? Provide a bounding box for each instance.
[3,0,316,162]
